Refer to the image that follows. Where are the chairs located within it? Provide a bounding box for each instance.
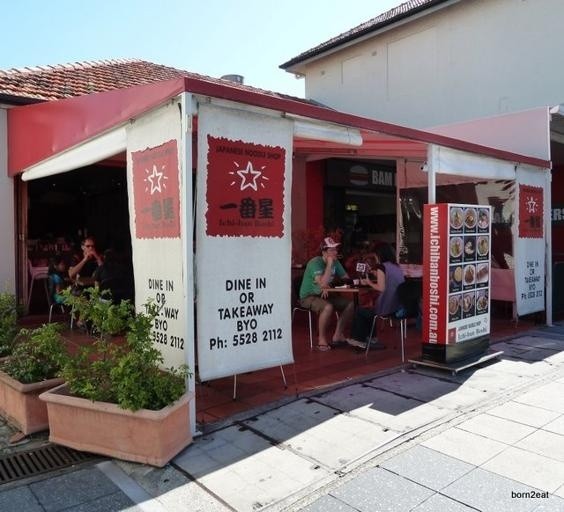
[292,267,420,364]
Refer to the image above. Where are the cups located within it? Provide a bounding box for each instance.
[353,279,360,285]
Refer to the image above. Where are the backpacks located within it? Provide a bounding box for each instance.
[391,282,420,319]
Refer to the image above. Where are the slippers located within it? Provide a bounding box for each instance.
[316,344,331,352]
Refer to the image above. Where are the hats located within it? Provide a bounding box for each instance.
[320,237,342,250]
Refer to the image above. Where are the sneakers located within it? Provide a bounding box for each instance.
[346,337,377,348]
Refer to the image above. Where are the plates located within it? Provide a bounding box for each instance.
[450,207,489,316]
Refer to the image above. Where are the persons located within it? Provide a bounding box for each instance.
[67,234,102,276]
[348,242,408,350]
[93,246,128,289]
[46,254,85,328]
[298,237,354,351]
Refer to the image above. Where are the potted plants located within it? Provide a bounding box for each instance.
[1,281,195,469]
